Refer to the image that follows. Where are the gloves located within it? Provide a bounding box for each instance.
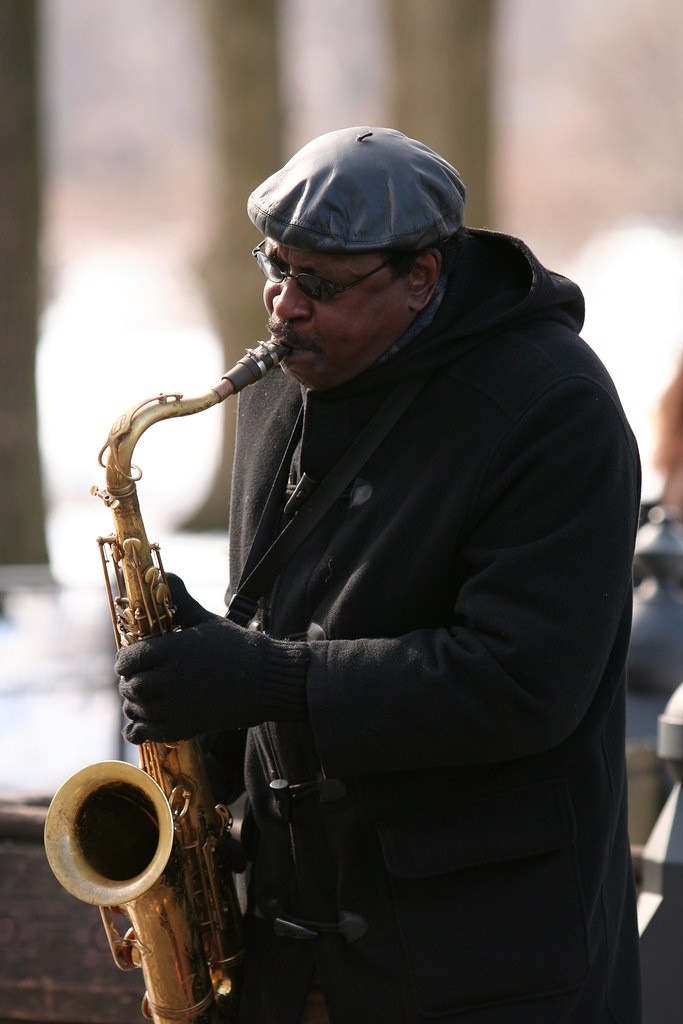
[114,572,309,745]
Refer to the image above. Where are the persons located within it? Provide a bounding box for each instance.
[114,124,644,1024]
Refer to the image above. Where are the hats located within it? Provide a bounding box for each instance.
[247,126,467,255]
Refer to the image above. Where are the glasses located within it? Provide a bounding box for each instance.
[251,240,385,299]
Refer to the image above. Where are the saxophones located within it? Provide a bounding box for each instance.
[39,334,298,1024]
